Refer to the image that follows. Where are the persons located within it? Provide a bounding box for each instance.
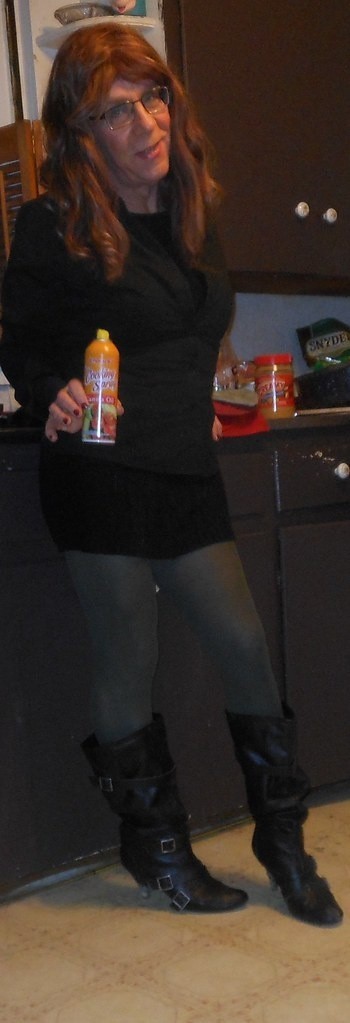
[0,23,344,928]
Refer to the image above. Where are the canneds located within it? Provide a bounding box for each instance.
[253,354,296,419]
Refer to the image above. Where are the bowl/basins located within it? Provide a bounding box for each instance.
[54,3,115,26]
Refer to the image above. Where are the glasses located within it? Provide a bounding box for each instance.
[89,84,169,132]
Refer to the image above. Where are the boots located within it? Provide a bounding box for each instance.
[225,702,344,926]
[80,710,249,912]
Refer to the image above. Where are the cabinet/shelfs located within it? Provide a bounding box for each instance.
[179,0,350,279]
[0,426,350,905]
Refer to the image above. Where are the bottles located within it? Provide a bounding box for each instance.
[81,327,120,447]
